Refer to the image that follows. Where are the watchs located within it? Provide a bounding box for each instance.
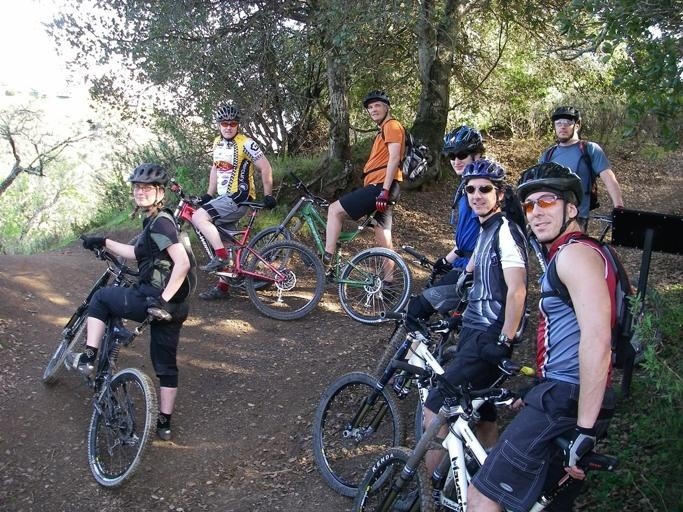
[497,333,513,344]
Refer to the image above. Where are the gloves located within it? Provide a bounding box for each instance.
[455,270,475,303]
[264,193,278,211]
[478,335,513,371]
[197,194,212,205]
[376,189,390,214]
[82,237,107,251]
[434,258,453,278]
[142,295,175,311]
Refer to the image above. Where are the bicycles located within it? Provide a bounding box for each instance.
[167,175,327,322]
[310,215,631,511]
[41,229,160,491]
[234,169,414,326]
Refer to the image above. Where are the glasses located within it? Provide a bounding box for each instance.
[555,120,576,128]
[448,151,478,162]
[133,184,159,193]
[463,185,499,194]
[368,104,386,111]
[220,122,237,128]
[521,194,561,215]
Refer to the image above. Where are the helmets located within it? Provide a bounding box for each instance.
[127,162,170,188]
[362,90,392,108]
[215,105,243,123]
[462,160,505,185]
[552,106,583,127]
[442,125,483,155]
[515,161,582,208]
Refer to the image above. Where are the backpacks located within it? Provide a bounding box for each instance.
[381,119,433,184]
[147,206,197,305]
[451,170,526,235]
[546,236,637,397]
[545,139,601,212]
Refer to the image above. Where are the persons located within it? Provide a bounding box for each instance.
[188,102,277,301]
[539,106,624,235]
[317,89,406,303]
[62,162,198,442]
[389,122,527,400]
[463,161,635,512]
[392,160,529,512]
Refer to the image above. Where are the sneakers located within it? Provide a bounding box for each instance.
[199,285,233,301]
[200,256,234,272]
[372,280,395,304]
[393,490,438,512]
[316,251,341,270]
[154,411,173,441]
[66,353,94,377]
